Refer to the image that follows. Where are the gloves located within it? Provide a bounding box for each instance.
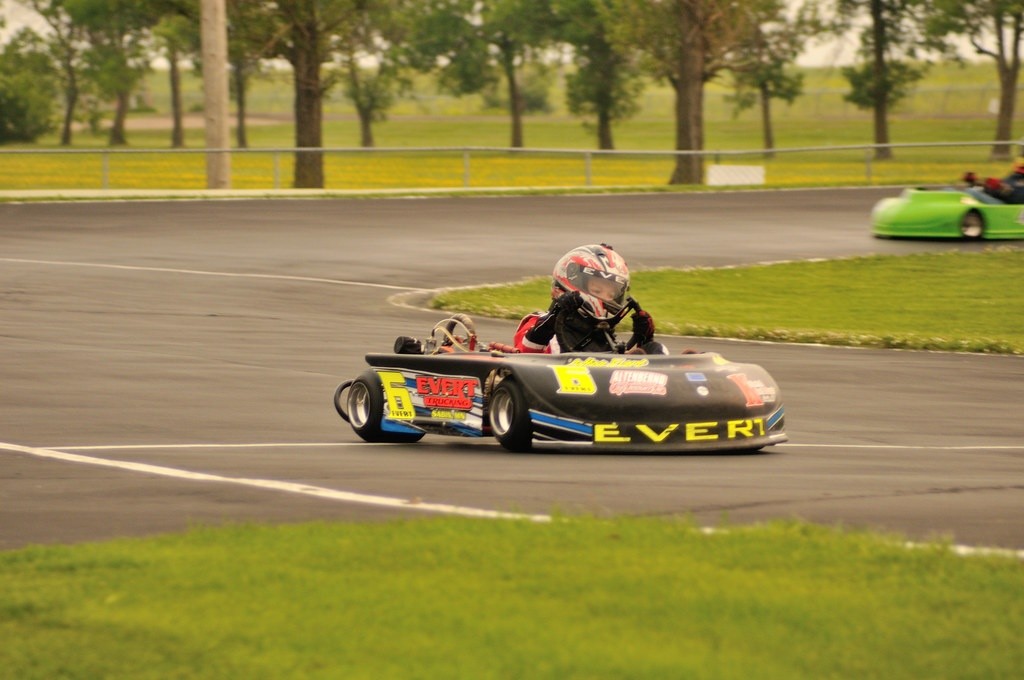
[548,290,584,322]
[985,177,1005,195]
[630,309,655,346]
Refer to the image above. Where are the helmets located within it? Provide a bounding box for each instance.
[552,244,629,320]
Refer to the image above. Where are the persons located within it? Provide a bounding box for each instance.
[960,136,1024,206]
[516,244,655,356]
